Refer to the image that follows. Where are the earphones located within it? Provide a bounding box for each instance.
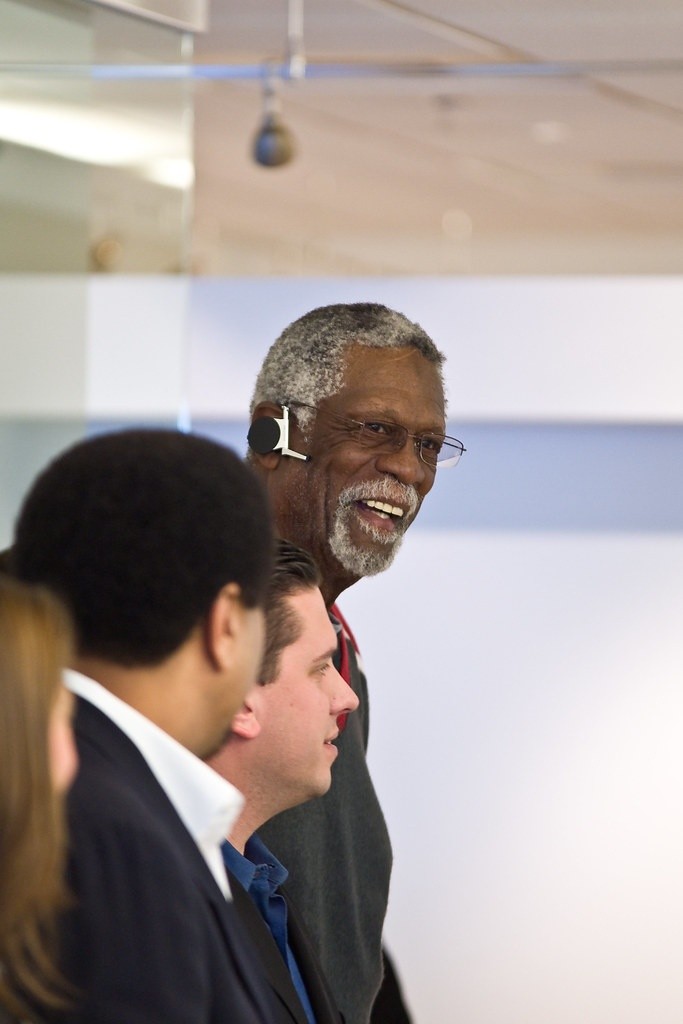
[248,401,311,464]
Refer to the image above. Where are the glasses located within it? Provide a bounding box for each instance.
[275,396,467,469]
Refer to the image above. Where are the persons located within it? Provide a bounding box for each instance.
[246,303,447,1024]
[0,428,360,1024]
